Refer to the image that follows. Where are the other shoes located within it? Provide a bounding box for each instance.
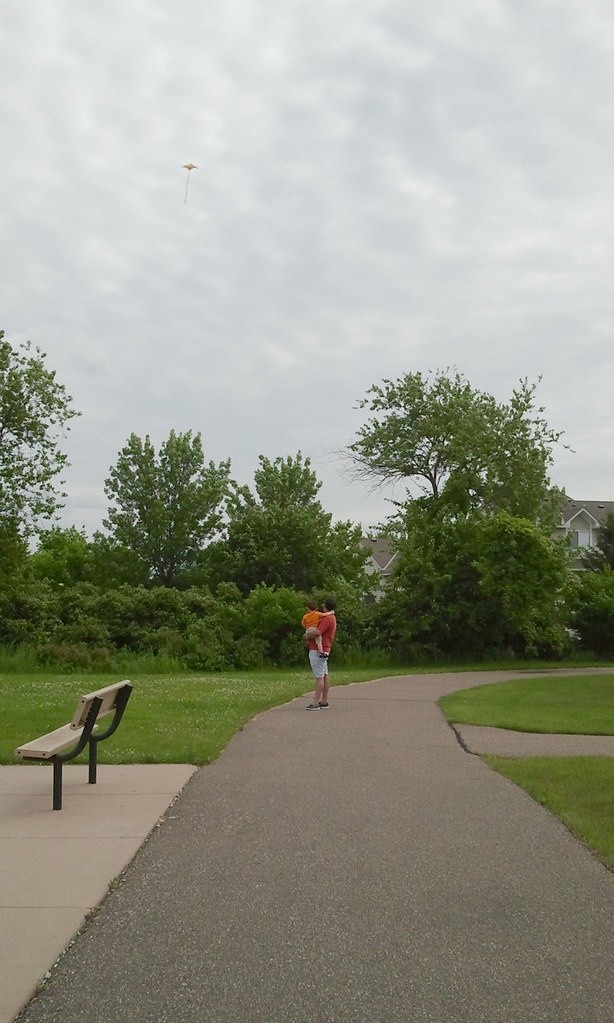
[306,702,320,710]
[318,702,329,708]
[318,651,329,658]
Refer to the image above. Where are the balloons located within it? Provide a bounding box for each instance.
[179,163,200,171]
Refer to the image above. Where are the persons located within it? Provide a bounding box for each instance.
[300,603,335,658]
[308,598,336,711]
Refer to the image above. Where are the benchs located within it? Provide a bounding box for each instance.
[14,680,134,811]
